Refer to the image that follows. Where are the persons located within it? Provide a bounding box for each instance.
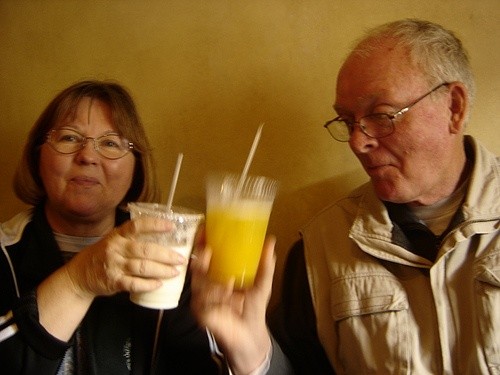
[190,19,500,375]
[0,77,234,375]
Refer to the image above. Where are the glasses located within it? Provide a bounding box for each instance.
[324,81,448,142]
[41,127,134,160]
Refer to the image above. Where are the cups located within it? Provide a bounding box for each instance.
[127,201,204,310]
[205,170,280,291]
